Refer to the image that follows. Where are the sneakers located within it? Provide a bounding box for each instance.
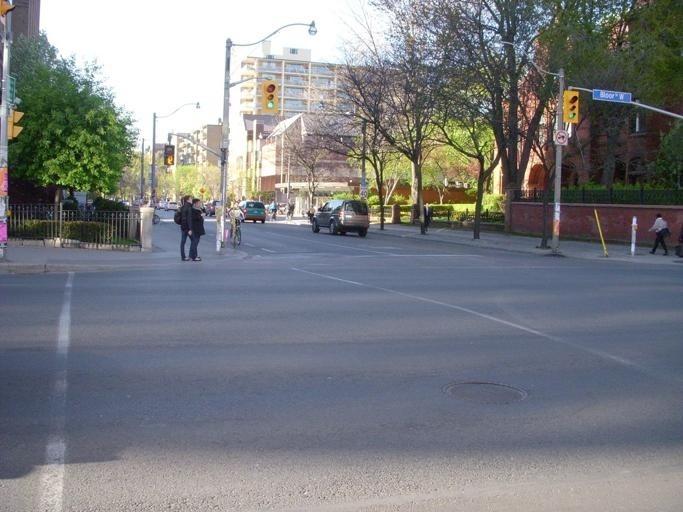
[649,251,655,255]
[182,257,201,261]
[663,254,668,256]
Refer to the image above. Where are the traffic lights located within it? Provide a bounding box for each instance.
[261,79,280,114]
[7,109,24,140]
[563,90,579,124]
[164,145,174,165]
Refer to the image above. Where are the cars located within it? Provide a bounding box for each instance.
[200,200,219,216]
[157,201,178,211]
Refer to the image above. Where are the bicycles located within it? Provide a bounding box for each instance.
[229,220,241,247]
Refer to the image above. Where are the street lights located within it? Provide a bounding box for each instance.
[220,21,317,248]
[151,102,200,196]
[490,41,564,255]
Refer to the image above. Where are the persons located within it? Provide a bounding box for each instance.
[227,201,245,240]
[187,198,206,260]
[647,212,671,256]
[268,200,278,214]
[179,195,192,261]
[423,202,433,226]
[308,205,315,221]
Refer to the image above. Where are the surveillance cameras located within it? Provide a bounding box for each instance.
[12,97,23,107]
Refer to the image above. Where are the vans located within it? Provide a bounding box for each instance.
[238,201,266,223]
[312,200,370,236]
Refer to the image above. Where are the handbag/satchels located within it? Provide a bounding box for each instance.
[661,228,669,237]
[174,212,181,225]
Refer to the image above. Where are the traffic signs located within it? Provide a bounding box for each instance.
[360,189,368,198]
